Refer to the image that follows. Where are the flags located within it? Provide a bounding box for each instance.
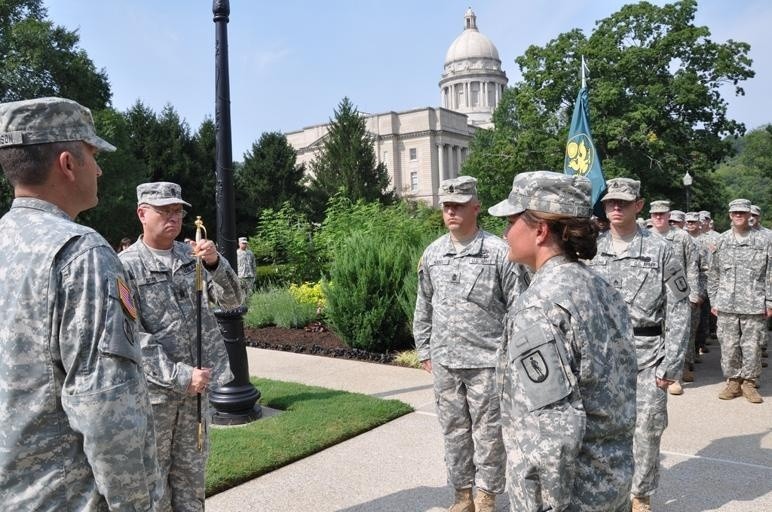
[564,56,607,209]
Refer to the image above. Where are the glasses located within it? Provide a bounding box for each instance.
[442,204,466,212]
[136,205,188,219]
[605,199,638,206]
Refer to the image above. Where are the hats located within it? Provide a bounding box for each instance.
[600,177,642,201]
[648,200,671,214]
[437,174,479,206]
[685,211,700,221]
[645,219,654,227]
[0,95,118,154]
[750,205,761,216]
[669,210,686,223]
[238,236,249,244]
[136,181,194,209]
[698,210,712,221]
[728,198,752,213]
[486,170,594,219]
[699,215,707,221]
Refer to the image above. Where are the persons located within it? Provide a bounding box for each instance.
[578,178,692,510]
[591,214,610,234]
[706,198,772,403]
[668,210,700,381]
[647,200,700,395]
[488,170,638,511]
[237,237,256,297]
[685,210,711,382]
[114,181,245,511]
[748,205,771,368]
[1,96,164,511]
[412,176,531,511]
[701,211,722,354]
[118,238,130,253]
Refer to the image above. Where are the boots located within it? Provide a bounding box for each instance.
[740,379,762,403]
[701,345,710,354]
[473,487,496,512]
[718,377,743,400]
[705,335,713,345]
[690,364,698,370]
[668,379,683,395]
[683,367,694,382]
[726,376,742,385]
[710,329,718,339]
[448,488,476,512]
[630,496,651,511]
[696,358,704,363]
[754,376,761,388]
[761,358,769,367]
[760,346,769,357]
